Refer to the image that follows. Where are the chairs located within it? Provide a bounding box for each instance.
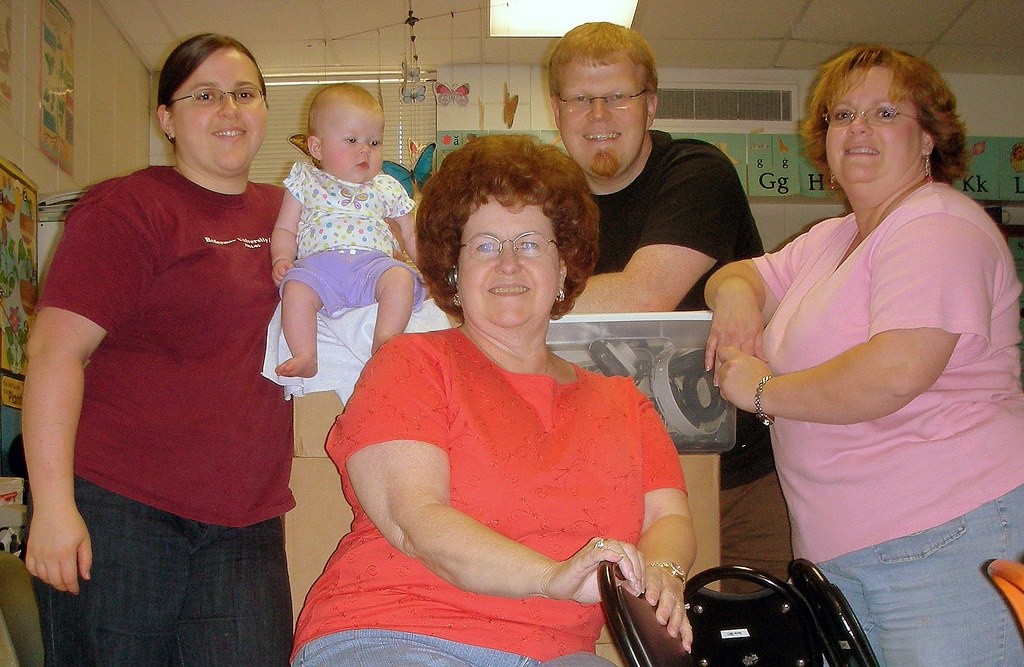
[594,557,882,667]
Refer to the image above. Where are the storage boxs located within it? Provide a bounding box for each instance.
[551,310,738,451]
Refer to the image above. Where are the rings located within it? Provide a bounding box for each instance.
[594,538,605,550]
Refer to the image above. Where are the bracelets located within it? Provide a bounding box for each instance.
[754,375,775,425]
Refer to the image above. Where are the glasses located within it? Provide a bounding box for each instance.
[169,86,265,110]
[556,86,649,115]
[457,232,557,258]
[823,107,921,128]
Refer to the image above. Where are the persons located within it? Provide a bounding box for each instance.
[704,43,1024,667]
[287,132,697,667]
[269,84,434,378]
[545,21,798,596]
[19,34,298,667]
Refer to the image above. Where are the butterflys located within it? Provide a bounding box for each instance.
[288,134,320,168]
[408,138,427,167]
[402,63,421,83]
[383,143,437,198]
[401,86,426,104]
[501,81,520,130]
[434,82,471,106]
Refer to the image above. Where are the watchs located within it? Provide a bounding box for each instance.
[646,561,686,594]
